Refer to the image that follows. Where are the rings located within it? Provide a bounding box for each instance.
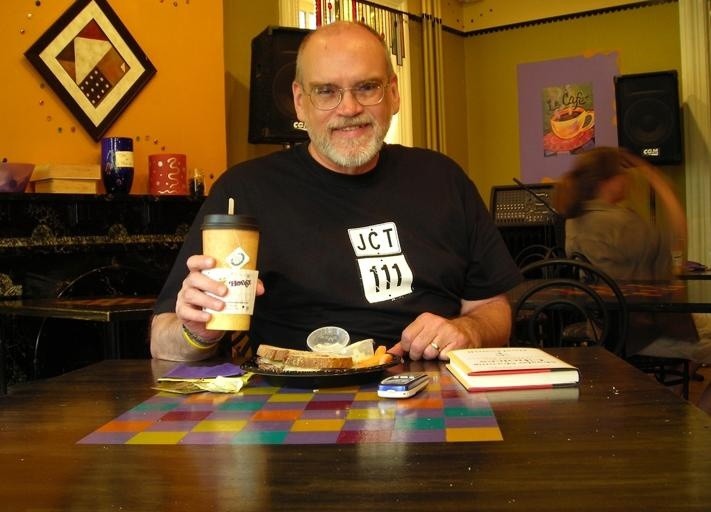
[429,342,440,350]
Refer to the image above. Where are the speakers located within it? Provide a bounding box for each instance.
[614,70,683,167]
[489,182,597,347]
[249,25,316,144]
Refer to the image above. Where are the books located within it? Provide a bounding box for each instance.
[446,346,583,394]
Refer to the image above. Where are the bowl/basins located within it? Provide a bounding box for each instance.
[0,162,37,192]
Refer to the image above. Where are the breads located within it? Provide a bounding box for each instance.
[256,343,353,372]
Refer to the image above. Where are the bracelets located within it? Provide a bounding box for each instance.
[181,325,226,350]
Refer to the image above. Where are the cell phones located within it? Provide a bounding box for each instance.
[377,372,429,398]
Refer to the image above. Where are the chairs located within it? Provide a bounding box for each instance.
[503,255,632,355]
[625,309,698,400]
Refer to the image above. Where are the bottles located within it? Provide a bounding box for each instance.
[201,214,262,332]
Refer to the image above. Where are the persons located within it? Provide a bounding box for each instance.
[149,19,524,363]
[552,146,702,375]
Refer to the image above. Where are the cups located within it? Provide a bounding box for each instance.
[147,153,188,197]
[550,107,594,140]
[102,137,136,196]
[189,168,205,195]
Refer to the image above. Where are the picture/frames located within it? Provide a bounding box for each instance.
[21,0,157,143]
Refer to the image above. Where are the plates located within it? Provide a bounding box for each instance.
[240,352,401,387]
[544,130,594,153]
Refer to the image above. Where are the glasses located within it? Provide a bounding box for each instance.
[310,81,386,111]
